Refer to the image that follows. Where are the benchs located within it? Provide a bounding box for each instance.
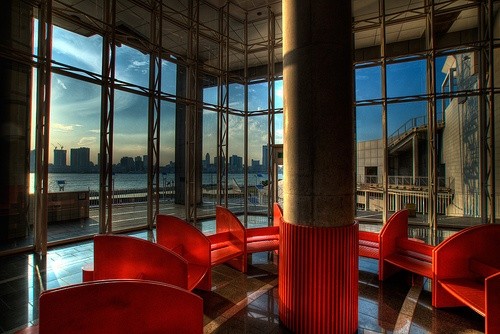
[13,202,283,334]
[358,208,500,334]
